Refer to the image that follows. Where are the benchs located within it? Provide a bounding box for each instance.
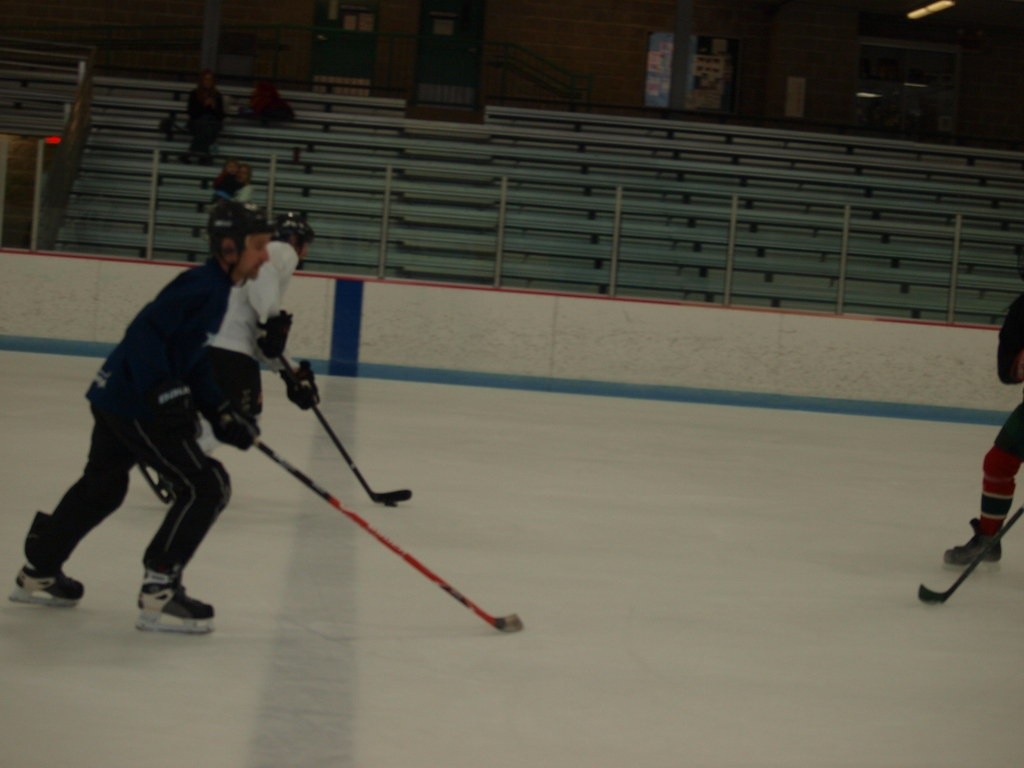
[1,64,1024,324]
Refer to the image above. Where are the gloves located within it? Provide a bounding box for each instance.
[157,377,199,435]
[205,396,259,449]
[280,359,320,410]
[257,309,294,358]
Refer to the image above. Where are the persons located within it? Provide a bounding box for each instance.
[211,161,253,203]
[943,246,1024,566]
[7,199,273,633]
[177,69,225,164]
[138,210,320,500]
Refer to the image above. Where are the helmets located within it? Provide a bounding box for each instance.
[273,212,315,243]
[208,200,275,249]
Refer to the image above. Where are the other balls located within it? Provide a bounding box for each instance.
[384,499,399,508]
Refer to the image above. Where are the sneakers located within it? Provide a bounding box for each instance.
[135,569,213,634]
[941,518,1005,570]
[8,540,84,606]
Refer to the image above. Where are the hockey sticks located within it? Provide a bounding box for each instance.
[253,316,413,507]
[251,439,523,632]
[918,502,1024,607]
